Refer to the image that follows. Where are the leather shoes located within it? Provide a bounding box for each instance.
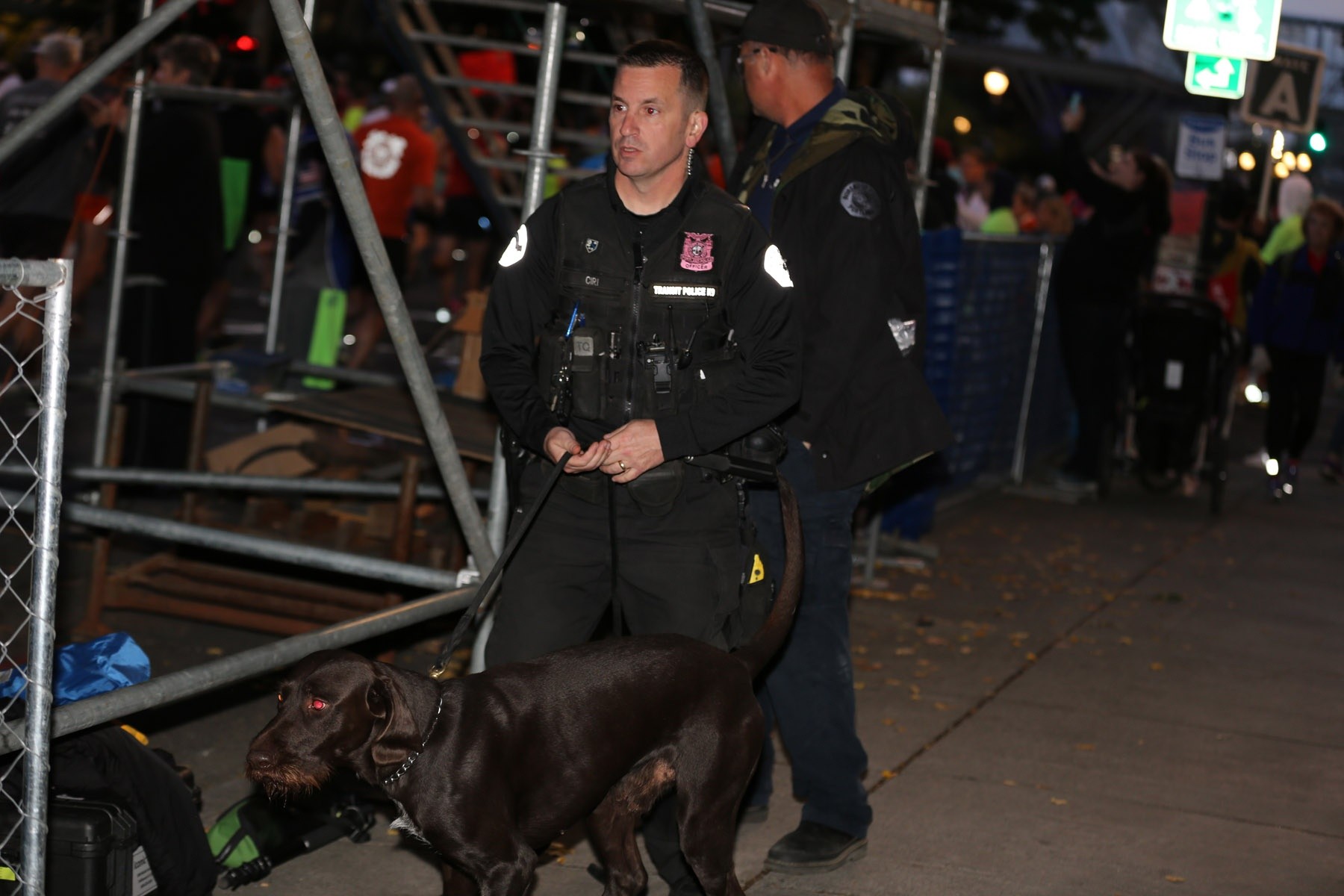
[740,798,767,824]
[762,821,868,874]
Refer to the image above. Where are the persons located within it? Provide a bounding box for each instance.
[477,39,803,893]
[0,2,613,399]
[892,92,1344,510]
[714,1,919,873]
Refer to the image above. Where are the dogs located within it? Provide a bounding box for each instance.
[246,463,805,896]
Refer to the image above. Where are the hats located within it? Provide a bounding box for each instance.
[727,0,834,55]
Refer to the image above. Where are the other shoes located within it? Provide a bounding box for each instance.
[1055,470,1101,492]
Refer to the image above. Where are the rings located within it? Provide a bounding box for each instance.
[618,460,626,471]
